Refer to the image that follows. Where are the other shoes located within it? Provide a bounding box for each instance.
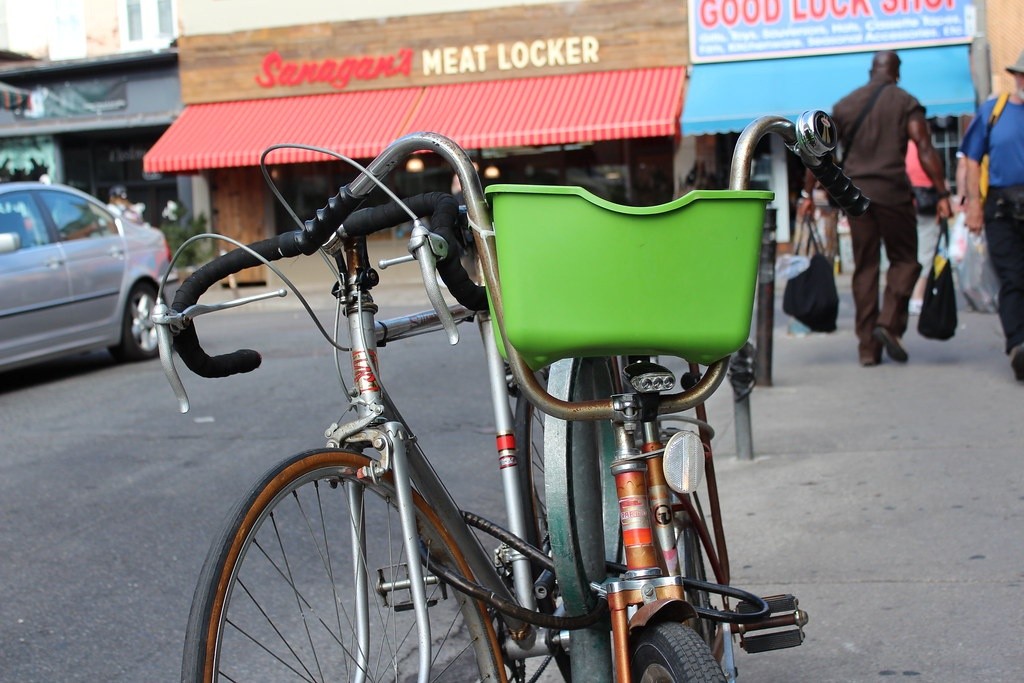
[860,353,879,365]
[874,327,908,361]
[1010,343,1024,381]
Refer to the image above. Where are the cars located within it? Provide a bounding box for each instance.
[0,180,182,373]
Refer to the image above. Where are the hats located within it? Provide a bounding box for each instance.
[1005,51,1024,75]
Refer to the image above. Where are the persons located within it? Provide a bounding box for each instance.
[799,51,954,368]
[65,185,142,239]
[958,57,1024,384]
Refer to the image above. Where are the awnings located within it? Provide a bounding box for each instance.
[143,42,977,173]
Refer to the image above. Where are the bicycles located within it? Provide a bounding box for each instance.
[291,110,875,683]
[150,140,626,683]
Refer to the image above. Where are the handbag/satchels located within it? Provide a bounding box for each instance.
[958,226,1001,315]
[783,215,840,335]
[917,218,959,340]
